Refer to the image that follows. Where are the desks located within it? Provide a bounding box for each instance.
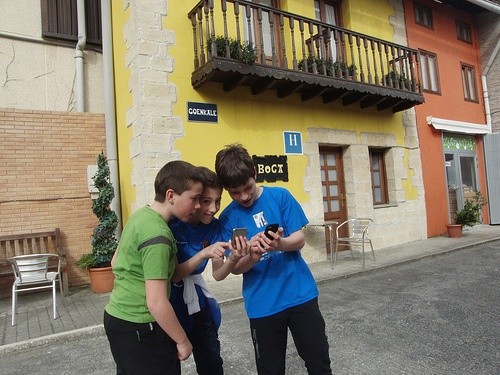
[308,222,338,264]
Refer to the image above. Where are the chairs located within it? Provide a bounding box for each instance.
[335,218,375,267]
[9,254,60,326]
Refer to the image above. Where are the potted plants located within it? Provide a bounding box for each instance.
[77,151,118,293]
[446,192,487,237]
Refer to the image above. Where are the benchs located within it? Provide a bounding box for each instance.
[0,227,68,296]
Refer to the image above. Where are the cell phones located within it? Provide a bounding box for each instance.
[232,228,246,249]
[261,224,279,248]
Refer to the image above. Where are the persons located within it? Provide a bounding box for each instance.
[103,161,206,375]
[167,166,251,375]
[214,140,333,375]
